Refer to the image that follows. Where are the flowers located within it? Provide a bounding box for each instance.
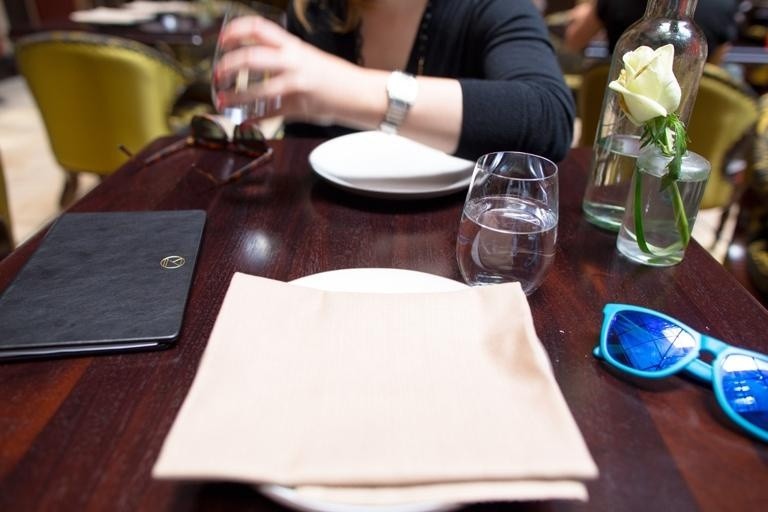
[605,43,693,255]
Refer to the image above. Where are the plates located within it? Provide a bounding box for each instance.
[253,268,479,509]
[309,129,491,195]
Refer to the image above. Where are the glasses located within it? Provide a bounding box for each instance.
[593,303,767,441]
[118,115,275,185]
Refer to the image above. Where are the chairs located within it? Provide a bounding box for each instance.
[574,56,762,240]
[13,30,199,208]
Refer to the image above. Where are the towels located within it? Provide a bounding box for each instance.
[151,271,600,508]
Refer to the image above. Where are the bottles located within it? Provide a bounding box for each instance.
[581,0,709,233]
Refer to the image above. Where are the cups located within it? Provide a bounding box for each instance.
[208,12,288,123]
[457,151,560,299]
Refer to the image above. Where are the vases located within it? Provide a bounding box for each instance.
[614,149,714,268]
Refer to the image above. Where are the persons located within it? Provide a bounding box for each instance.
[213,0,577,164]
[564,0,739,60]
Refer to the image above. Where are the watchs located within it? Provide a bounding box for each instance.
[379,69,417,132]
